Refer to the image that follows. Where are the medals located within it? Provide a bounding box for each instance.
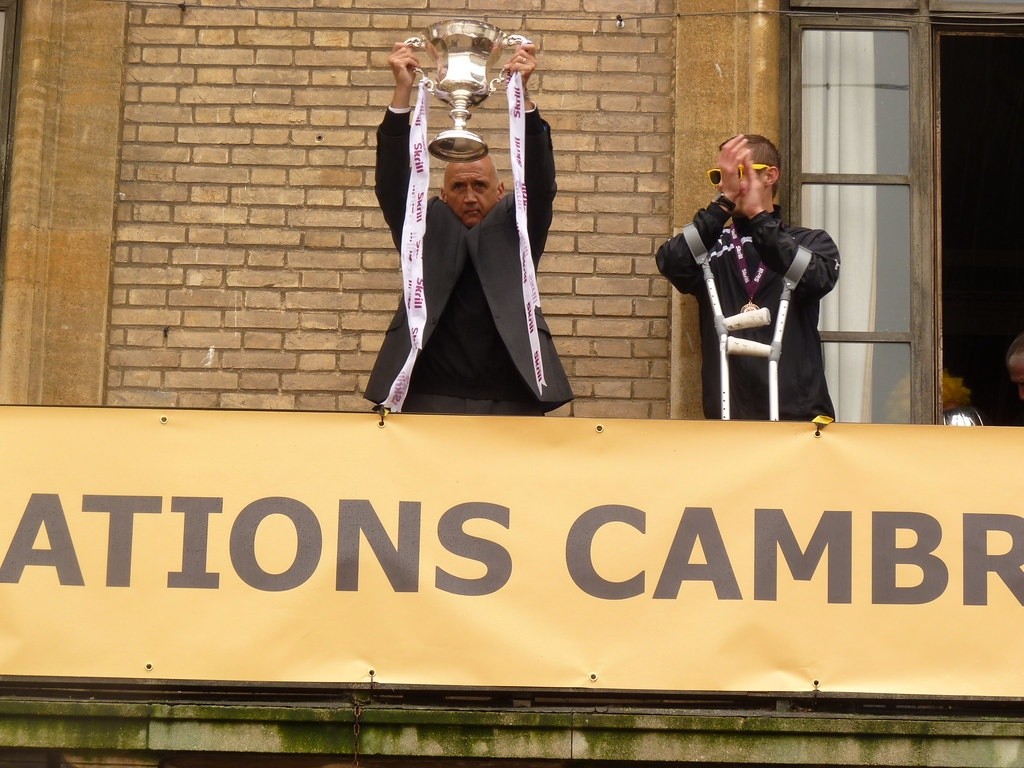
[740,303,760,314]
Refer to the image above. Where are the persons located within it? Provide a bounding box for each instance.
[361,41,575,418]
[655,134,840,423]
[1006,332,1024,402]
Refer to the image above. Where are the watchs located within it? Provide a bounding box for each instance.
[711,193,736,210]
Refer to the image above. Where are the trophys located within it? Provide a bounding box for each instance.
[401,18,531,164]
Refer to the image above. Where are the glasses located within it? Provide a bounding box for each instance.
[707,163,770,187]
